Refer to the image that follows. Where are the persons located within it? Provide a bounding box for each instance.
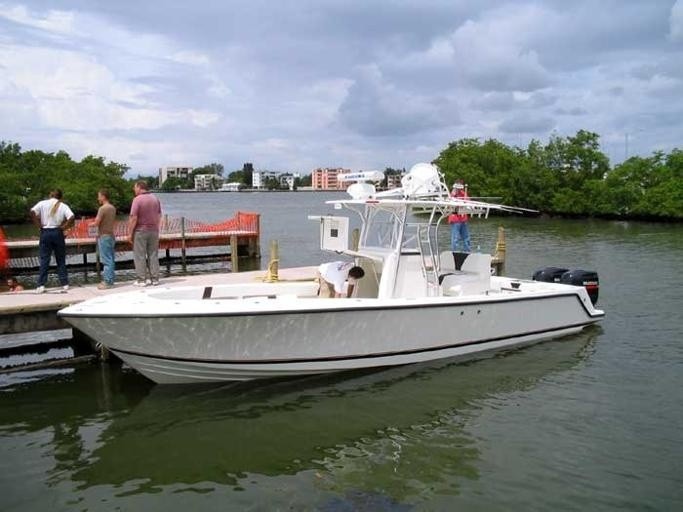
[125,180,164,286]
[28,188,74,293]
[5,277,23,292]
[87,189,116,289]
[315,259,364,297]
[444,178,474,253]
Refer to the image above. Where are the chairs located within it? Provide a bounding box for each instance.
[425,250,492,299]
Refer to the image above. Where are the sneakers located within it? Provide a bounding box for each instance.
[62,284,69,289]
[97,282,113,289]
[37,285,45,292]
[134,279,159,287]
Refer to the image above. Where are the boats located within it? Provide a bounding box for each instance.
[56,162,607,386]
